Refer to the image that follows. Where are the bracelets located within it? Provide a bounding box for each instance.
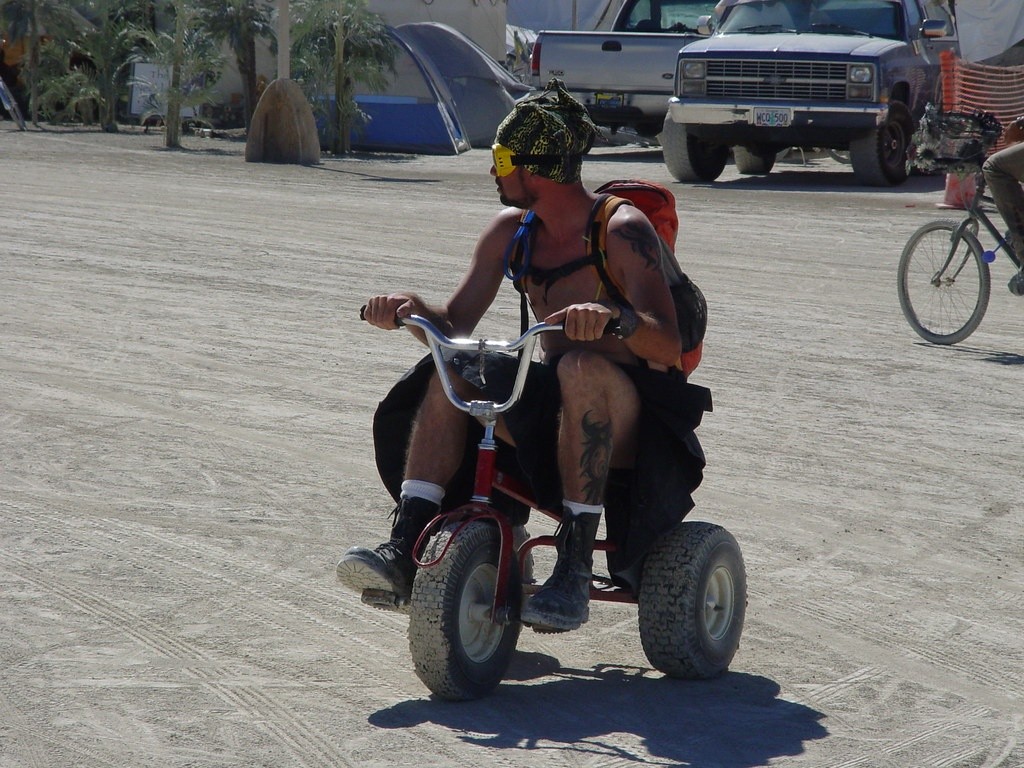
[1016,116,1024,131]
[612,305,638,339]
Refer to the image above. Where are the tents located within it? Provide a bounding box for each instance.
[306,22,537,155]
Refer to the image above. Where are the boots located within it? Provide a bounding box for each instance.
[520,505,602,632]
[337,494,442,596]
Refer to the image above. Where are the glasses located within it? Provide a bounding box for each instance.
[492,143,517,178]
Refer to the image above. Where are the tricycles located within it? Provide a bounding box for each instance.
[359,303,751,706]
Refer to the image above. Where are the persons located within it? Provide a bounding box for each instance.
[982,116,1024,296]
[337,77,686,633]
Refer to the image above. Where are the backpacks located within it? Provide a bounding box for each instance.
[503,179,707,381]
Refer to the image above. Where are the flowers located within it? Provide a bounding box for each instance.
[906,105,997,173]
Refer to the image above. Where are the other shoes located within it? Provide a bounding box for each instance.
[1008,271,1024,296]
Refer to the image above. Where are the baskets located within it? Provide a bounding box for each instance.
[916,101,1003,172]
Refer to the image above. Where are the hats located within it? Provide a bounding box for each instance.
[494,77,609,184]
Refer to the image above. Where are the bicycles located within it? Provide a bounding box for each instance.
[896,108,1024,346]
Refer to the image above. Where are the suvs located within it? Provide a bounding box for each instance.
[659,1,963,186]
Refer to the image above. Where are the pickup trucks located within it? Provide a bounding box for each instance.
[528,1,720,156]
